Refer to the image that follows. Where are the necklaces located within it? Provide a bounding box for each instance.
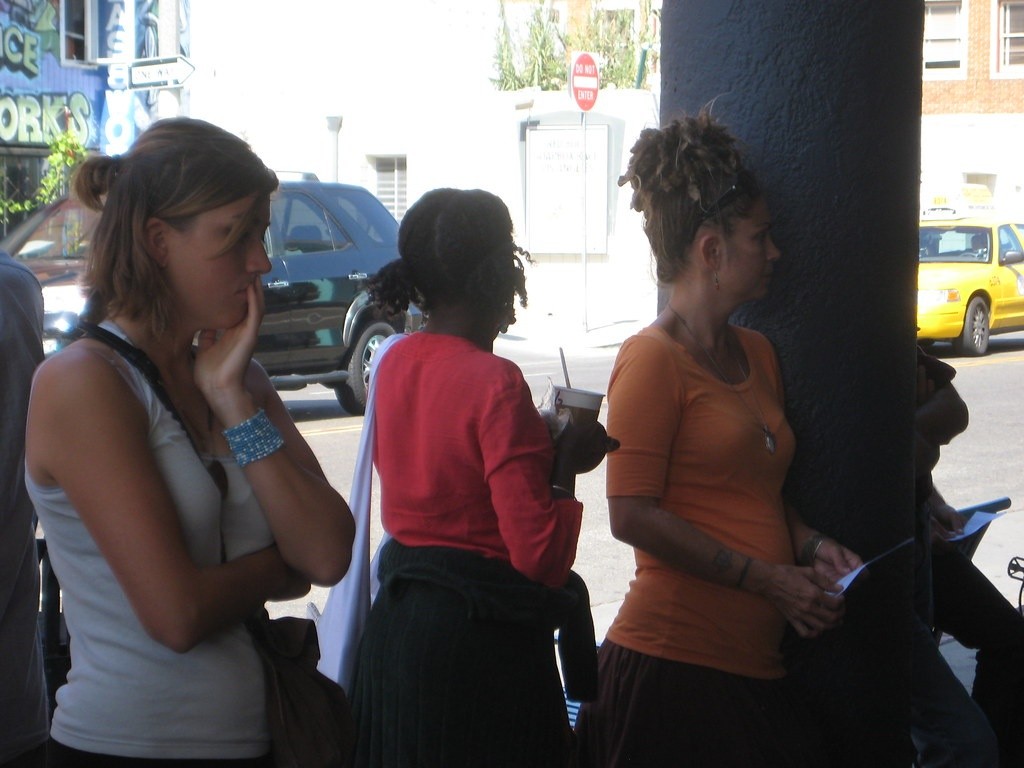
[169,398,231,567]
[668,303,776,453]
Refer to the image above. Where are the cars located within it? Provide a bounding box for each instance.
[917,182,1024,358]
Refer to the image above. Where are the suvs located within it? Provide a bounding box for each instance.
[248,172,423,418]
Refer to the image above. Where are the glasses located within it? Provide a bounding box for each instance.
[686,168,761,244]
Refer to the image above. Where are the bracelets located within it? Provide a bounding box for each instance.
[551,484,577,502]
[220,411,286,466]
[800,530,829,566]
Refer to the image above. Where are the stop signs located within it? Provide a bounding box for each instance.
[569,50,601,113]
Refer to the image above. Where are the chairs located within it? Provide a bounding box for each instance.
[284,226,322,253]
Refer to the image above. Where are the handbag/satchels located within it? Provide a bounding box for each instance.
[243,618,356,768]
[305,535,393,701]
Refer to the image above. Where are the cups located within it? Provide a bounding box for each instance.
[554,386,606,421]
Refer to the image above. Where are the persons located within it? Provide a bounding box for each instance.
[0,110,1024,768]
